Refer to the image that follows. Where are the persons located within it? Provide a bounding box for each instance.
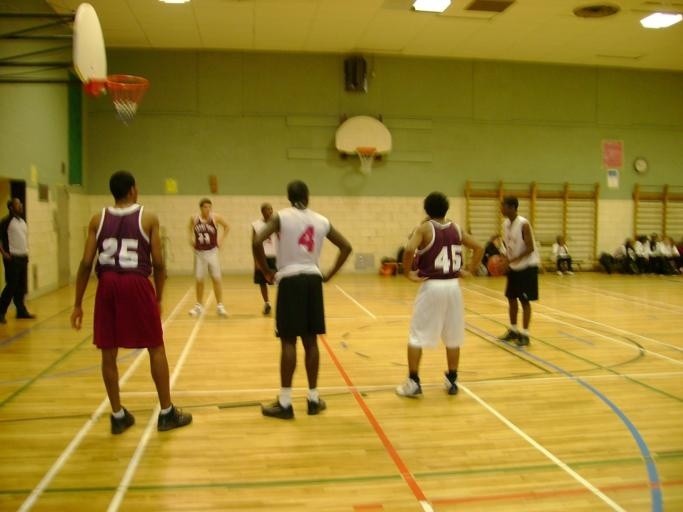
[251,177,352,420]
[552,234,575,278]
[494,196,540,347]
[249,199,282,315]
[0,196,36,324]
[393,189,485,398]
[610,228,682,277]
[67,171,194,434]
[186,198,230,319]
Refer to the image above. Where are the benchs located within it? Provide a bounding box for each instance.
[539,260,602,272]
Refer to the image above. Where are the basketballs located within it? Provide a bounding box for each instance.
[486,254,511,277]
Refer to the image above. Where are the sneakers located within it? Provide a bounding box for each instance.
[445,372,457,395]
[500,329,529,346]
[111,409,134,433]
[189,305,227,316]
[262,304,272,314]
[262,401,294,418]
[396,379,422,395]
[157,405,191,431]
[308,399,326,414]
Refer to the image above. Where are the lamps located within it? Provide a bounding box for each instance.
[639,12,683,29]
[412,0,452,13]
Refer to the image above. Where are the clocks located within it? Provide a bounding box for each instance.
[633,157,649,174]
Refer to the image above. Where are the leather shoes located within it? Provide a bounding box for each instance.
[0,314,36,324]
